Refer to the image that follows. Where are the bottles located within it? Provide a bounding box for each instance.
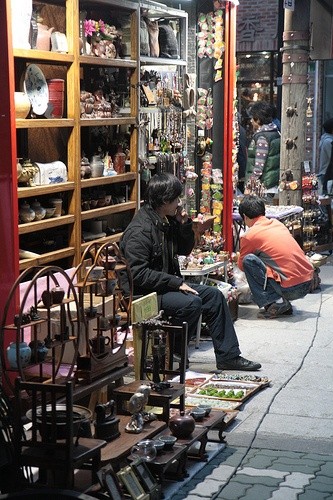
[13,92,30,119]
[6,339,49,368]
[20,200,47,222]
[114,145,126,174]
[90,155,104,176]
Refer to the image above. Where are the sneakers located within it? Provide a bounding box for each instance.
[257,297,293,319]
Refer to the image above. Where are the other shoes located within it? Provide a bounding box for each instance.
[216,356,261,371]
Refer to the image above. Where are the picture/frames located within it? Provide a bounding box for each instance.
[132,456,158,491]
[119,466,146,500]
[102,469,124,500]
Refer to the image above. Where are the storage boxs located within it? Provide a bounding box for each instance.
[31,291,115,363]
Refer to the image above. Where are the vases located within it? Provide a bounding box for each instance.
[113,145,126,174]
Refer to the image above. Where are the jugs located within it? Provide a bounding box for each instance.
[131,439,156,462]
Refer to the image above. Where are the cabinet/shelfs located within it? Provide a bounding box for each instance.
[0,267,80,416]
[67,241,134,384]
[0,0,189,280]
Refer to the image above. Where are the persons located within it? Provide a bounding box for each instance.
[320,120,333,229]
[244,103,280,195]
[237,112,247,178]
[237,194,315,319]
[116,172,261,371]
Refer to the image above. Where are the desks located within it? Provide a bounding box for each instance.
[8,365,133,404]
[180,259,230,348]
[233,196,333,253]
[58,409,227,482]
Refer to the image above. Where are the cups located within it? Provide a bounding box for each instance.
[193,404,212,423]
[45,199,63,218]
[154,435,177,456]
[50,285,64,303]
[45,79,63,119]
[83,307,122,326]
[41,290,53,306]
[85,266,117,292]
[82,194,112,210]
[91,219,108,234]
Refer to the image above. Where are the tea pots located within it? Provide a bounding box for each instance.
[33,23,55,51]
[99,253,119,271]
[169,411,196,438]
[89,333,111,354]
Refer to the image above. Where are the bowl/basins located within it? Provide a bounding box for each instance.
[19,64,51,115]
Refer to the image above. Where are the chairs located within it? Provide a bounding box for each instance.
[12,373,108,490]
[114,321,190,421]
[90,247,177,380]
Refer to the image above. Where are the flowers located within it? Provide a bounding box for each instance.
[83,17,119,42]
